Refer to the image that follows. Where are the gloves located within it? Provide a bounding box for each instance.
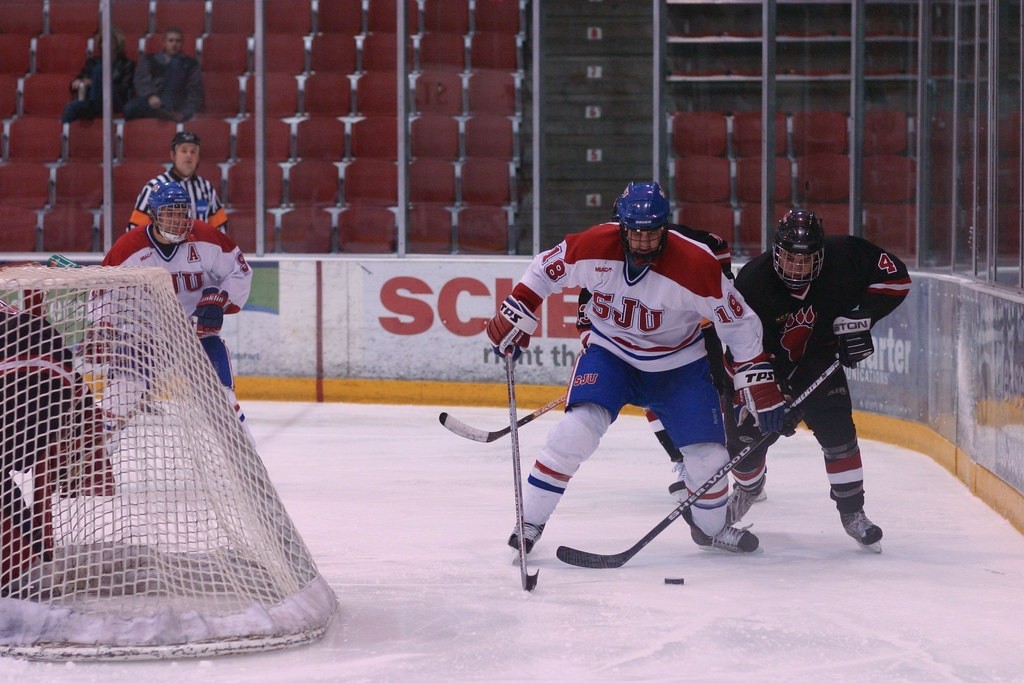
[834,321,874,366]
[186,286,228,338]
[484,296,541,363]
[734,363,794,436]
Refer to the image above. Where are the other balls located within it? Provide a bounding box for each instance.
[663,576,685,585]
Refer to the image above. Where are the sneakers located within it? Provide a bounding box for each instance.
[668,463,686,503]
[679,504,760,554]
[508,516,545,563]
[831,488,882,555]
[722,479,770,524]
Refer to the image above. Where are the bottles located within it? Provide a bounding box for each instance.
[79,83,86,102]
[47,254,81,269]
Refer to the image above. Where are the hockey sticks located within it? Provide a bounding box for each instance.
[438,392,568,444]
[555,360,842,569]
[504,345,539,591]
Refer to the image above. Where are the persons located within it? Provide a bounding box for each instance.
[1,301,94,588]
[76,182,257,450]
[61,27,205,122]
[486,182,912,554]
[126,132,229,235]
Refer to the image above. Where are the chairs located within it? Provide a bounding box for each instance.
[662,0,1024,267]
[0,0,530,254]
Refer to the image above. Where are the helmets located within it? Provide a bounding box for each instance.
[146,181,192,240]
[170,133,200,146]
[771,208,828,288]
[616,181,671,263]
